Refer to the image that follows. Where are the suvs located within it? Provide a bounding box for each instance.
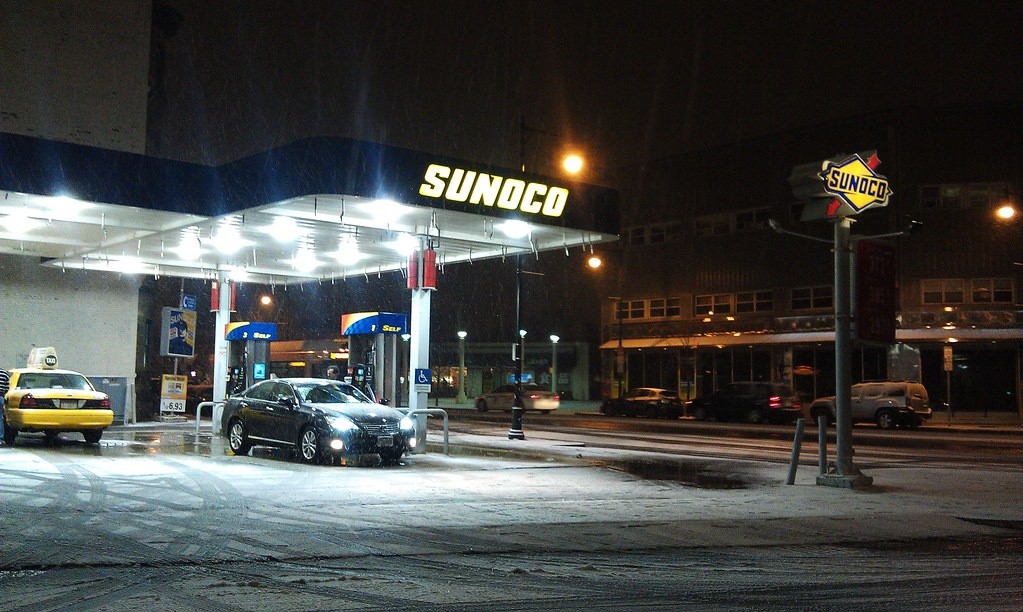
[810,381,933,429]
[693,381,802,424]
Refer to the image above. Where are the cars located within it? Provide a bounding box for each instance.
[2,347,114,443]
[600,388,684,419]
[221,377,416,465]
[474,383,560,414]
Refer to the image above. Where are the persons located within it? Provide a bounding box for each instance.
[0,368,10,446]
[326,365,347,393]
[169,320,193,356]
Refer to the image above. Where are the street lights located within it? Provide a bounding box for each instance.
[401,333,410,401]
[508,120,586,441]
[587,245,626,398]
[519,329,528,373]
[550,334,560,392]
[455,330,467,404]
